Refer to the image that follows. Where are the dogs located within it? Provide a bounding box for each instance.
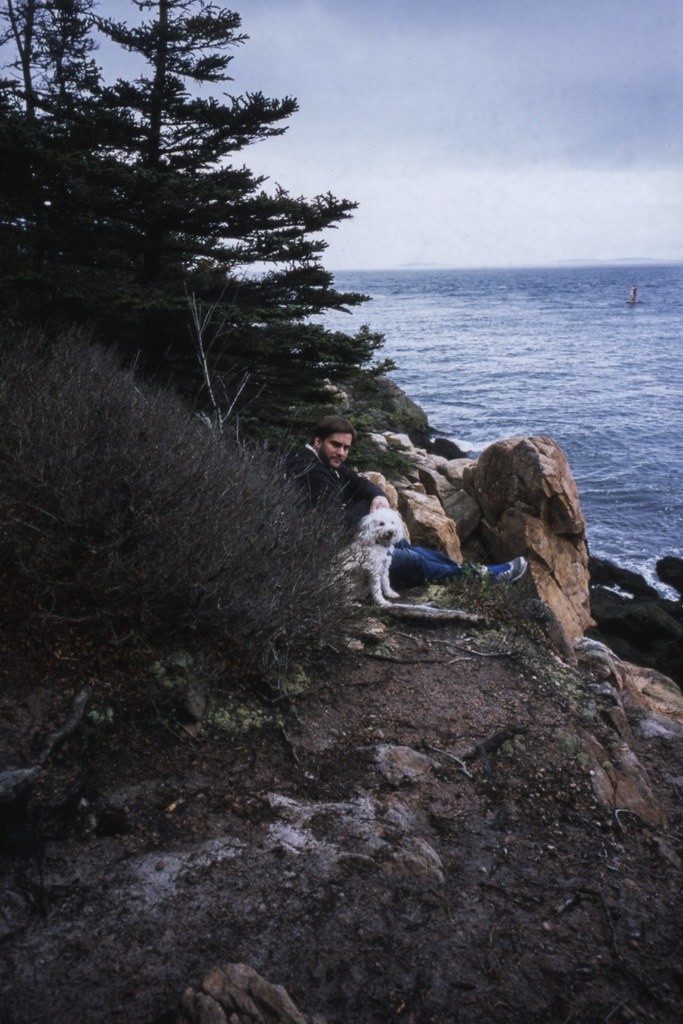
[331,509,404,607]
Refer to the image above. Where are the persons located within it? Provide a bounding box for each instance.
[284,415,527,590]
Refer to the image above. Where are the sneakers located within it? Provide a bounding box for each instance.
[488,556,527,591]
